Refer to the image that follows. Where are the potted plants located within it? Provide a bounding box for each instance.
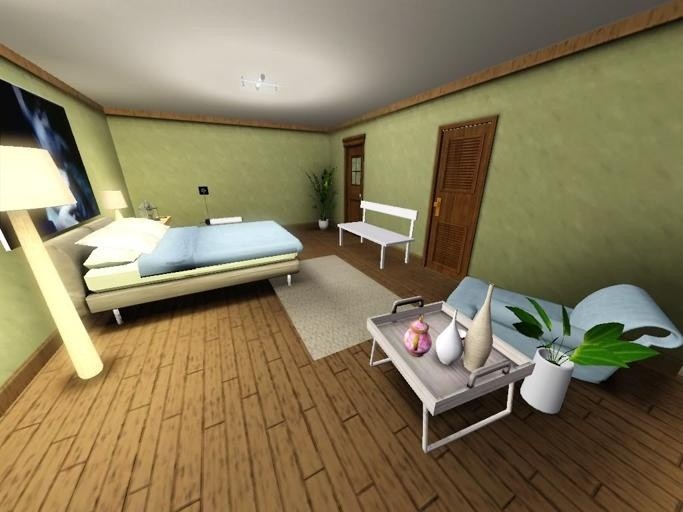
[302,166,340,230]
[504,296,662,416]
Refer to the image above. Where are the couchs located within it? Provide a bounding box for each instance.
[445,276,683,386]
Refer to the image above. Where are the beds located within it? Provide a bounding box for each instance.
[84,219,304,326]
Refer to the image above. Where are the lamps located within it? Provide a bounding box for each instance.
[0,144,105,381]
[103,190,129,220]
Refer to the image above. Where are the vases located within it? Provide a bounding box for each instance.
[435,309,464,367]
[462,283,493,373]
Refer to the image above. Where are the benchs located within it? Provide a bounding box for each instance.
[336,200,419,270]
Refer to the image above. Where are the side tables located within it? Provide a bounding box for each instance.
[366,295,536,454]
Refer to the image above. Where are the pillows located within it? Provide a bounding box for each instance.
[74,217,170,270]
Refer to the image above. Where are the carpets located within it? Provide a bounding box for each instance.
[268,255,416,362]
